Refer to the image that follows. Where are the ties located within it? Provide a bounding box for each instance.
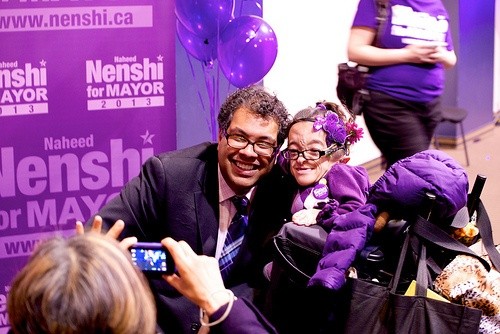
[219,196,253,288]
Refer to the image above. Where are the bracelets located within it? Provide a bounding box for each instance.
[199,289,235,327]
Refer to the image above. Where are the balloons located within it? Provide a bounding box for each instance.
[174,0,279,89]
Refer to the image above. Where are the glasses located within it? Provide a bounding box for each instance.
[224,127,281,158]
[281,143,339,160]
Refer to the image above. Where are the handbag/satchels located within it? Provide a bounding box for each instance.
[263,228,483,334]
[336,62,371,116]
[410,191,500,334]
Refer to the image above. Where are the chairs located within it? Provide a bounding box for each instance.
[434,107,469,167]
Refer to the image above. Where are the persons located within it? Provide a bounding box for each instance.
[82,85,301,334]
[5,216,266,334]
[348,1,457,171]
[265,101,374,295]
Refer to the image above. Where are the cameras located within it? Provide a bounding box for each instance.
[129,241,175,275]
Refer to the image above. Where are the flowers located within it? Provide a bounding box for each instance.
[344,122,364,145]
